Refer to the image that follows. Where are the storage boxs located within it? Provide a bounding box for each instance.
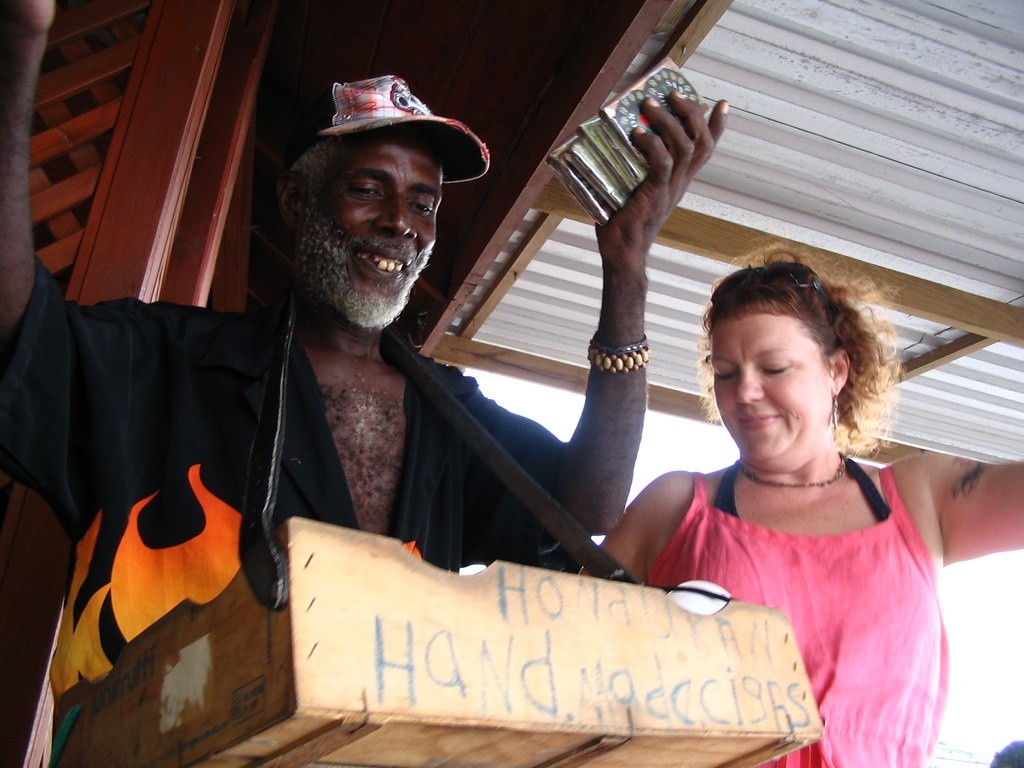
[54,517,825,768]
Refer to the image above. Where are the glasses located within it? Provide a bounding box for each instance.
[710,258,838,322]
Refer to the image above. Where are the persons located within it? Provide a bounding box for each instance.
[579,245,1023,768]
[0,0,727,697]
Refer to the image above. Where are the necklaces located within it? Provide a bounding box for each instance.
[739,451,846,487]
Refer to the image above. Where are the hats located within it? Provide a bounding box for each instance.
[284,72,490,184]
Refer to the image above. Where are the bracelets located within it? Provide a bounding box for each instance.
[586,333,650,374]
